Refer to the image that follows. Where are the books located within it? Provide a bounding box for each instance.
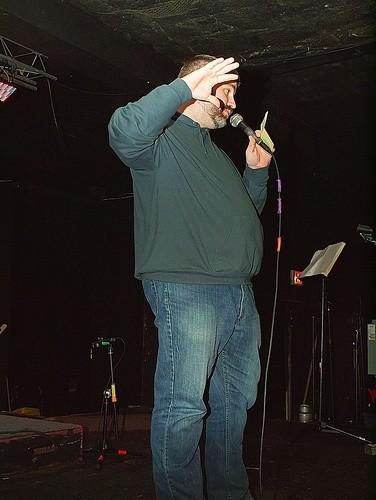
[298,241,346,278]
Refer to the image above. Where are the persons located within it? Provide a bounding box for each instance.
[108,55,273,500]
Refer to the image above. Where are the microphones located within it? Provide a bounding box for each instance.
[91,338,117,349]
[230,114,272,153]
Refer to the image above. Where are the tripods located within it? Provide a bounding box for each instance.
[288,241,373,446]
[83,344,147,460]
